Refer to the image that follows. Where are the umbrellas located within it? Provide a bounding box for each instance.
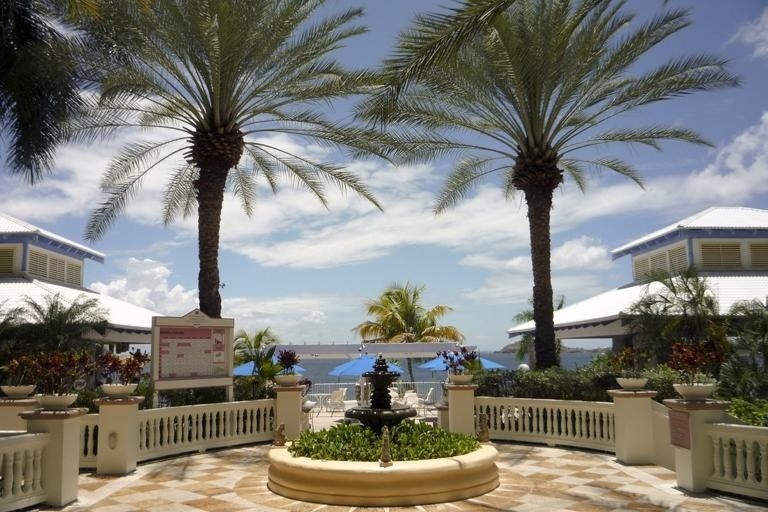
[327,351,404,399]
[232,353,308,399]
[429,352,507,372]
[417,349,463,369]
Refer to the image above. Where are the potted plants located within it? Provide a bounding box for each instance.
[0,353,43,399]
[34,348,95,409]
[100,348,150,398]
[668,342,718,401]
[612,344,649,391]
[273,348,304,388]
[436,344,478,384]
